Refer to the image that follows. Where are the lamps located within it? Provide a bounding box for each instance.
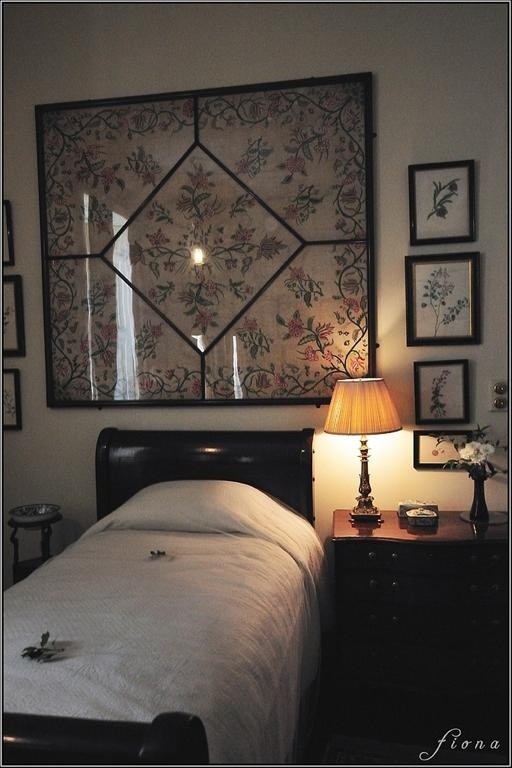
[324,377,403,524]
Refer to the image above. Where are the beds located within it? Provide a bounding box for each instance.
[4,427,335,764]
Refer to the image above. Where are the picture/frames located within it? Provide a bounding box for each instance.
[412,428,472,470]
[33,71,379,408]
[2,198,15,267]
[407,158,477,246]
[404,251,481,346]
[3,273,25,358]
[3,367,23,430]
[413,358,470,425]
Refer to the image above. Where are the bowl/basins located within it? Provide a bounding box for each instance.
[7,502,61,529]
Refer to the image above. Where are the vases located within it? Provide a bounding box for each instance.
[469,474,489,522]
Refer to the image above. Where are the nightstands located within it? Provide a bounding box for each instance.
[331,508,508,754]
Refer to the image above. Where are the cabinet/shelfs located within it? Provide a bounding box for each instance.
[7,512,65,585]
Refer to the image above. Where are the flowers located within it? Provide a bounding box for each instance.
[434,423,508,480]
